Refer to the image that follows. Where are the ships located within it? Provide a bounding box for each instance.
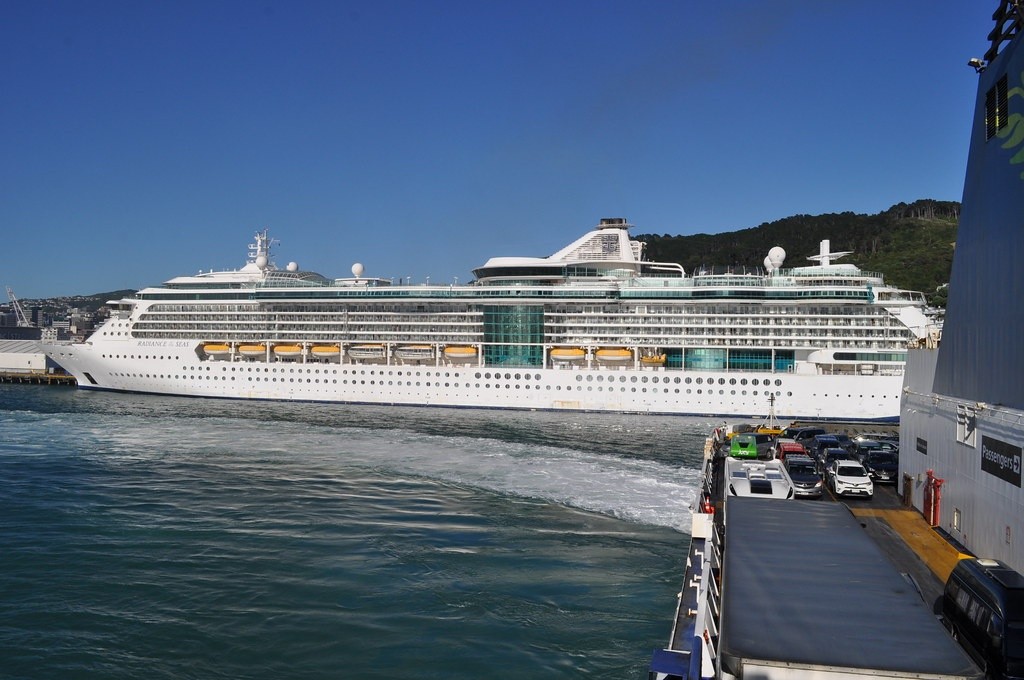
[37,228,942,425]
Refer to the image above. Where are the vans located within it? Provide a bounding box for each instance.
[718,433,774,459]
[771,426,827,449]
[942,556,1024,680]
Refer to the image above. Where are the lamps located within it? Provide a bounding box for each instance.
[902,386,910,393]
[975,402,985,408]
[967,57,985,73]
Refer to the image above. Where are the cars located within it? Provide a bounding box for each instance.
[775,438,824,498]
[851,433,900,485]
[811,434,874,501]
